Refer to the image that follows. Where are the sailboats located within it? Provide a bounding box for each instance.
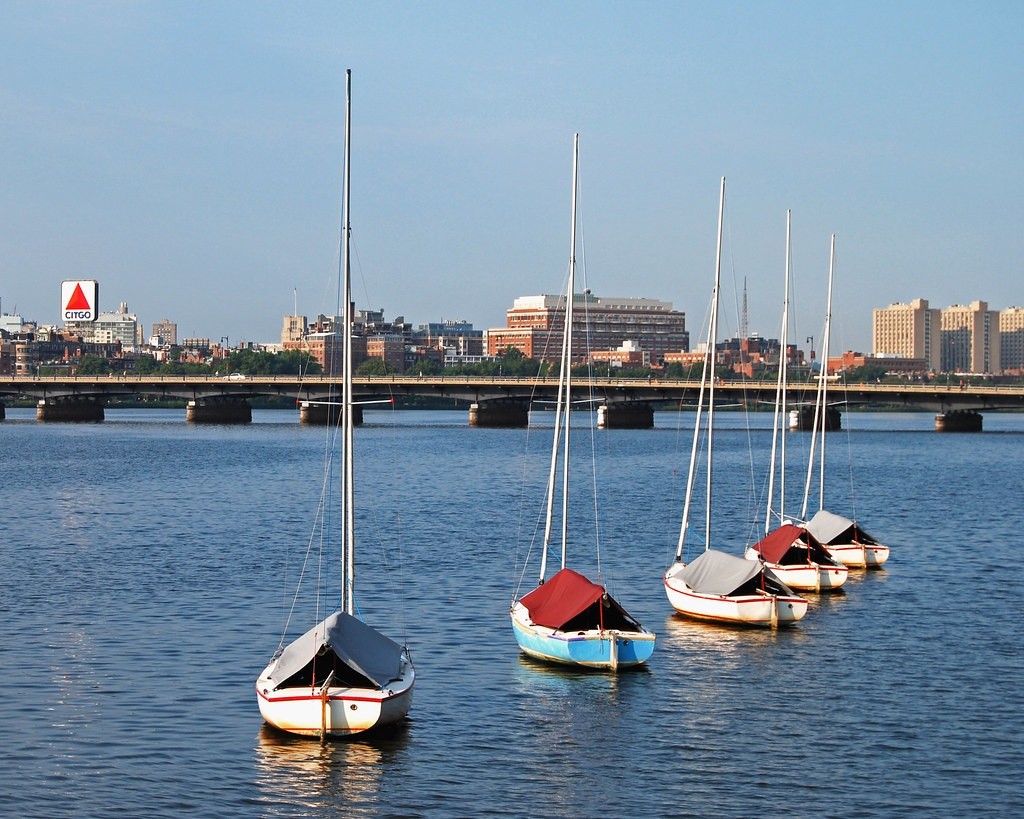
[790,232,890,570]
[663,175,807,629]
[511,132,656,673]
[256,70,415,746]
[744,210,848,593]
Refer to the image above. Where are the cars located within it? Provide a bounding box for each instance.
[223,373,245,380]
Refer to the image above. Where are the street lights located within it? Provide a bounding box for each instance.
[221,336,230,381]
[299,328,309,377]
[807,336,814,383]
